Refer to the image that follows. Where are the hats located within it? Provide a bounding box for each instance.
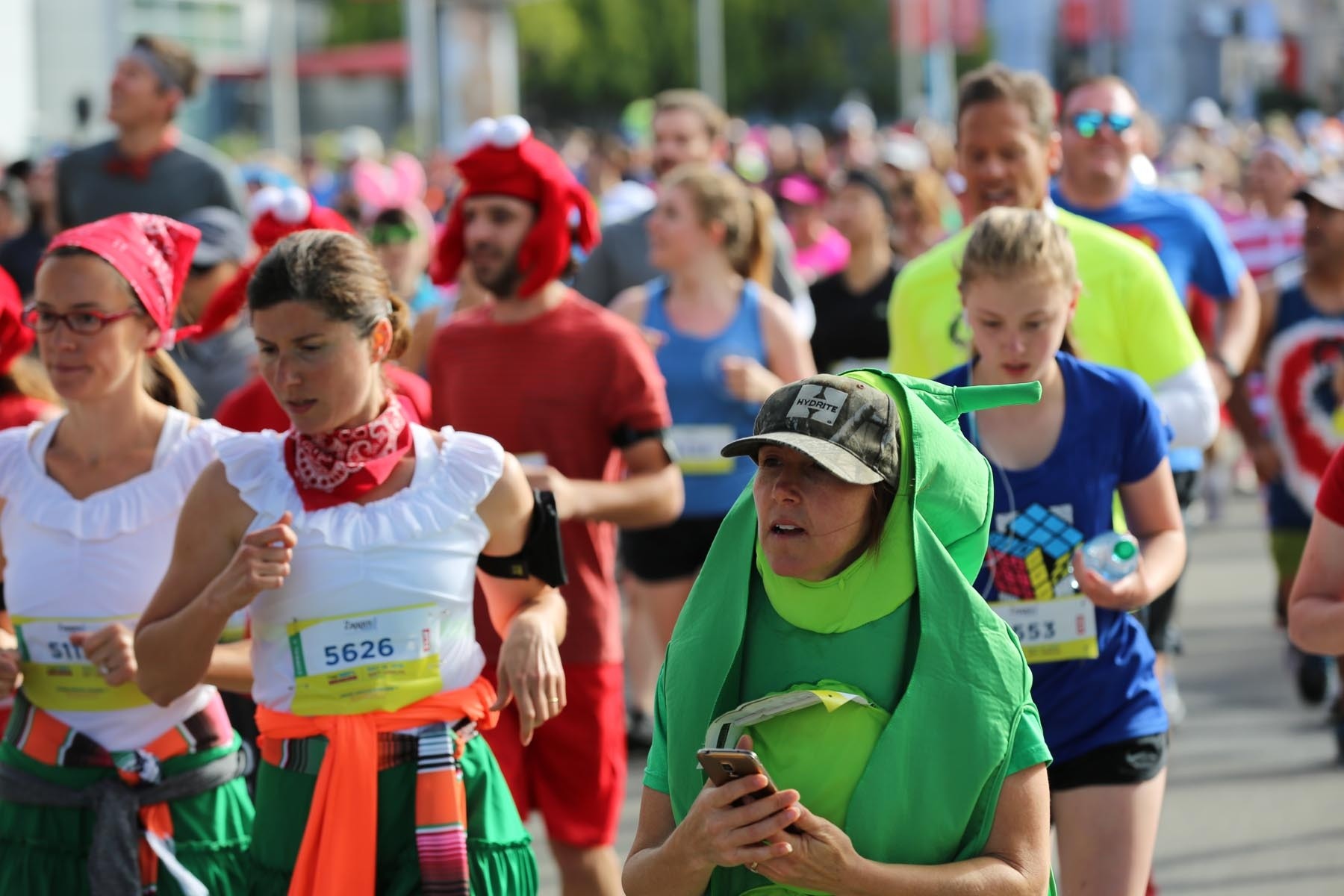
[1293,179,1344,215]
[721,375,900,489]
[179,207,256,267]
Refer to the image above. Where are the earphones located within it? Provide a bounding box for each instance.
[696,749,804,838]
[962,308,971,331]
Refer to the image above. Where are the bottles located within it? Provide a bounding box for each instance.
[1079,530,1139,580]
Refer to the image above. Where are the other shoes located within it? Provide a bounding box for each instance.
[1155,665,1185,729]
[627,707,652,751]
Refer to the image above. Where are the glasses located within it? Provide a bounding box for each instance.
[22,305,138,335]
[1065,109,1134,133]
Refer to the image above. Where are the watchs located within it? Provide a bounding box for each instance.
[1206,348,1242,380]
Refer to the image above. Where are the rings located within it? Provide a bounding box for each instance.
[99,662,110,676]
[99,663,108,675]
[750,861,758,872]
[547,697,559,703]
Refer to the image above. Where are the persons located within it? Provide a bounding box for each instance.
[923,206,1189,896]
[0,110,684,896]
[0,0,1344,767]
[623,366,1056,896]
